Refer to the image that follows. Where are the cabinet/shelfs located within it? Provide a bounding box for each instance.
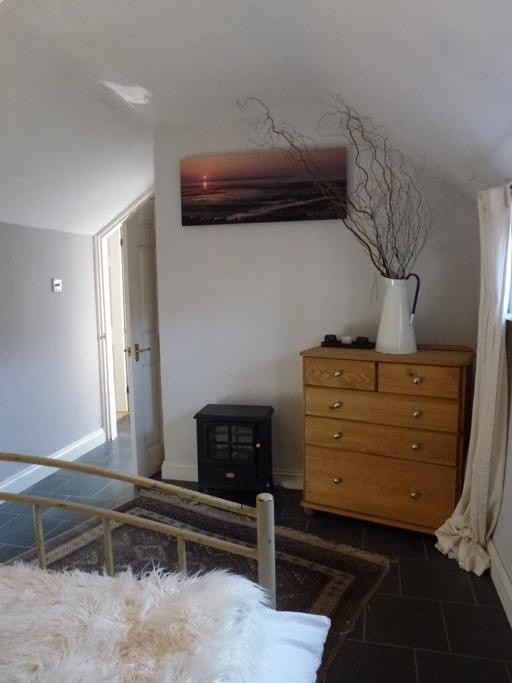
[300,345,476,536]
[193,404,273,493]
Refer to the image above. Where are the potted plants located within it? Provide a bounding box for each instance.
[237,88,435,354]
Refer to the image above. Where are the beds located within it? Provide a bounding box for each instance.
[0,452,333,683]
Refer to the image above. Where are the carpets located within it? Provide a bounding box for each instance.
[25,486,394,682]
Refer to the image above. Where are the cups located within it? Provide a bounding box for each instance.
[341,333,353,345]
